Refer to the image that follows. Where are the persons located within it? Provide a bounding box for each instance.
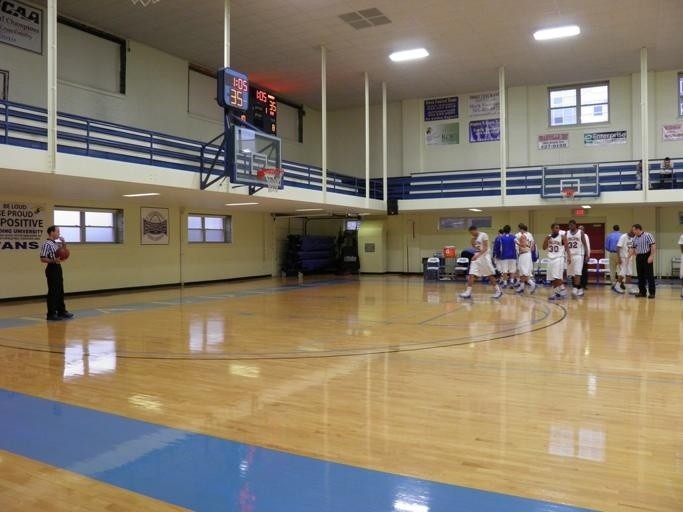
[635,160,642,190]
[660,157,676,188]
[47,320,65,383]
[678,234,683,300]
[456,220,657,298]
[458,297,655,364]
[40,225,73,320]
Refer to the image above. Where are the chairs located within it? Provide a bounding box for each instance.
[532,258,611,285]
[425,258,440,281]
[453,257,470,284]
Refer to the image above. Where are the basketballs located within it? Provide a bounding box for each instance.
[55,247,69,260]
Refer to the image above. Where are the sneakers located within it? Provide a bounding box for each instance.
[46,312,73,321]
[504,277,538,295]
[491,289,503,300]
[571,287,584,297]
[546,287,569,300]
[611,280,655,298]
[459,288,473,299]
